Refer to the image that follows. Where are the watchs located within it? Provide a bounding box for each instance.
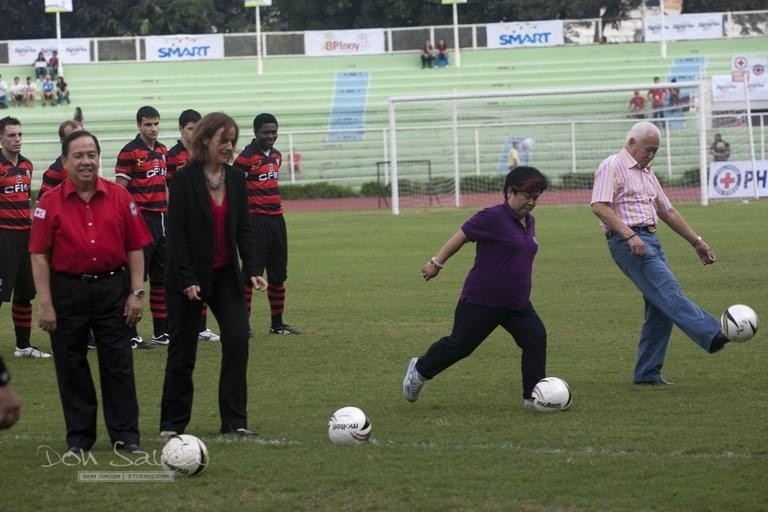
[131,287,147,298]
[692,235,701,246]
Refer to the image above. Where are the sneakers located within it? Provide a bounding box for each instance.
[403,356,426,402]
[269,324,303,336]
[13,346,52,362]
[523,398,534,407]
[152,332,171,344]
[198,329,221,341]
[129,334,153,354]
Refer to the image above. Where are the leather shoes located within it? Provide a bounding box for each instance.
[222,425,258,440]
[634,373,674,385]
[67,446,86,454]
[709,334,730,354]
[126,441,139,451]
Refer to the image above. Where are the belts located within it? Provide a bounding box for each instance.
[58,263,130,280]
[606,224,657,240]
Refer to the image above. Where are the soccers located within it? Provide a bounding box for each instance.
[329,407,372,445]
[722,304,759,344]
[716,141,726,153]
[531,376,573,412]
[161,434,209,479]
[522,138,536,152]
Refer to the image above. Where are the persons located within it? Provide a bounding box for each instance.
[591,122,731,385]
[648,76,668,128]
[31,52,50,82]
[666,78,681,114]
[53,76,70,105]
[402,166,547,405]
[159,113,268,442]
[47,50,59,80]
[29,131,154,456]
[23,76,36,108]
[506,137,520,169]
[39,74,56,106]
[0,357,21,430]
[420,39,434,68]
[166,109,221,342]
[286,148,302,174]
[711,133,730,161]
[0,117,53,359]
[435,39,451,69]
[10,76,26,106]
[628,89,645,119]
[115,106,169,349]
[0,74,9,107]
[36,121,97,202]
[232,113,299,337]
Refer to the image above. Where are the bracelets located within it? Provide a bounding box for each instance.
[430,258,444,269]
[623,233,638,240]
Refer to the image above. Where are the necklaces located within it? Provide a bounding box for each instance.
[204,167,226,191]
